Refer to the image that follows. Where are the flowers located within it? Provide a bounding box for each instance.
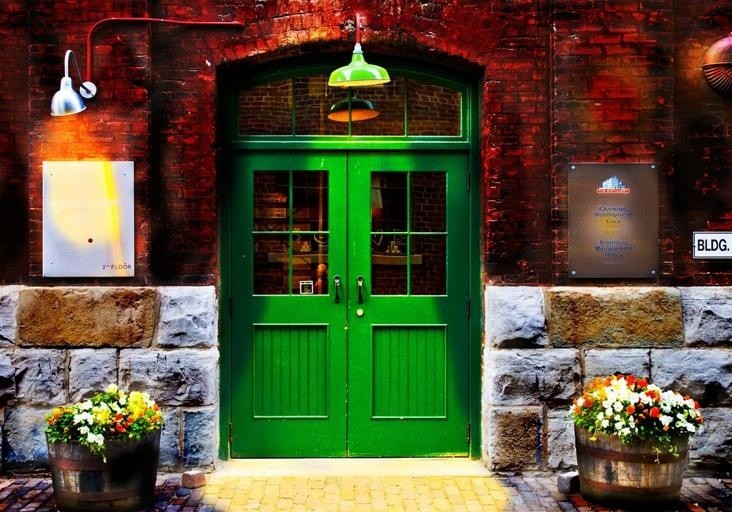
[41,385,168,463]
[561,374,707,454]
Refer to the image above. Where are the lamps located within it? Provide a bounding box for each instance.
[42,49,97,117]
[325,8,392,88]
[320,95,382,123]
